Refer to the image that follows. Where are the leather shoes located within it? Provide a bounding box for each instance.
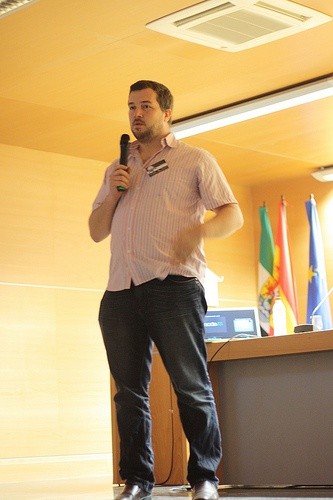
[115,482,152,500]
[193,480,219,500]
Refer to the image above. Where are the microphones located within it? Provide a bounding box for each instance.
[119,133,130,189]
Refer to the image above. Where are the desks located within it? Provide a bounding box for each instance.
[111,340,227,485]
[206,330,333,488]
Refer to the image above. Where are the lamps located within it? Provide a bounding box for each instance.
[169,76,333,140]
[312,166,333,182]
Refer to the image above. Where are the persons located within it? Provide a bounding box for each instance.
[89,79,242,500]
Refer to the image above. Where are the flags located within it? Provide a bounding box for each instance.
[304,200,332,331]
[268,200,299,337]
[258,206,275,338]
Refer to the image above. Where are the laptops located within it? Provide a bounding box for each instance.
[203,307,261,341]
